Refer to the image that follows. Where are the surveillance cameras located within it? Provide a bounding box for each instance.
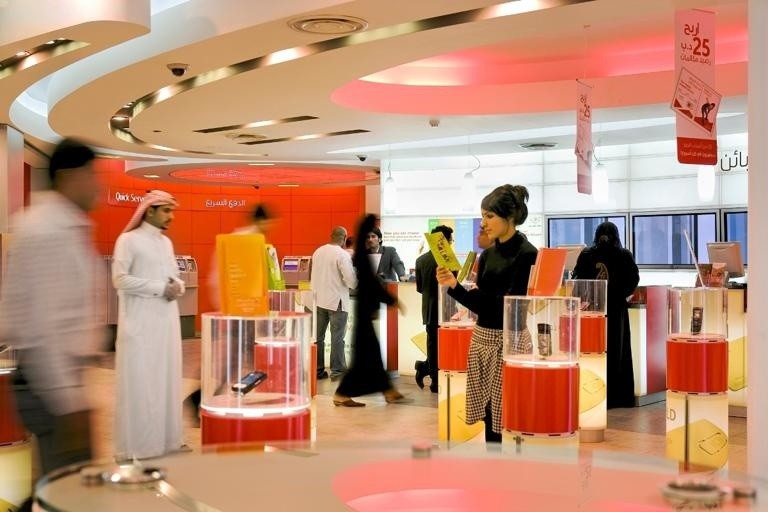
[358,155,368,161]
[167,64,189,76]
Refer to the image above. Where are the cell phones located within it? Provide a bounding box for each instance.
[537,322,552,357]
[580,301,590,310]
[451,308,468,320]
[274,321,285,337]
[232,371,267,395]
[691,307,703,334]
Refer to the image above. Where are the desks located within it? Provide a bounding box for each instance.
[35,442,764,512]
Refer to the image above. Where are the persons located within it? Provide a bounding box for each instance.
[366,226,410,282]
[573,221,640,410]
[343,237,357,259]
[308,226,360,383]
[435,184,539,452]
[1,138,113,477]
[183,203,275,428]
[415,225,458,393]
[468,217,496,282]
[332,213,416,407]
[111,190,193,461]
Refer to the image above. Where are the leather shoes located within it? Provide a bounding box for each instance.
[430,383,438,393]
[415,361,426,389]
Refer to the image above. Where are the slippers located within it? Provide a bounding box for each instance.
[334,400,365,407]
[386,392,405,403]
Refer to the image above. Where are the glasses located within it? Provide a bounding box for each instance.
[448,239,454,243]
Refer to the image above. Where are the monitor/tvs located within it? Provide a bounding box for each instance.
[706,242,745,287]
[557,243,586,278]
